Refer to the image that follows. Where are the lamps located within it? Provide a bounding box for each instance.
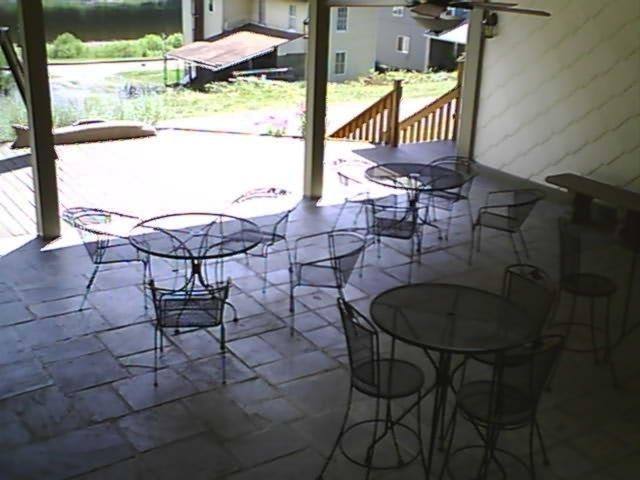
[411,13,467,37]
[480,13,496,37]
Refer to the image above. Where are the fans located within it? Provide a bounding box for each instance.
[331,0,551,19]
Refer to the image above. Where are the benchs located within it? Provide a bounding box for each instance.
[546,174,639,223]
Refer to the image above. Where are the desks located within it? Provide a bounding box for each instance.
[366,162,465,252]
[129,212,262,334]
[369,282,541,479]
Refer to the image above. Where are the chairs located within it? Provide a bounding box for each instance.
[290,229,366,337]
[547,214,617,366]
[148,276,230,387]
[415,155,481,242]
[445,263,556,442]
[62,207,154,311]
[471,188,546,264]
[359,198,423,285]
[318,297,429,480]
[220,188,299,294]
[333,172,397,232]
[440,333,566,480]
